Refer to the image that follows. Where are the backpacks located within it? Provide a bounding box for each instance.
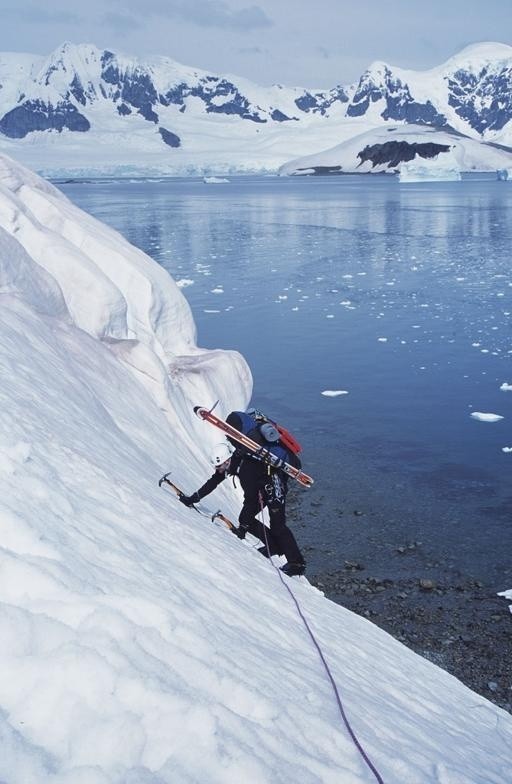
[225,411,302,469]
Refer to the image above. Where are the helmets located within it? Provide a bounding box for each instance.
[208,443,233,468]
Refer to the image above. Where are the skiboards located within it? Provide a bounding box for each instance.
[194,406,314,488]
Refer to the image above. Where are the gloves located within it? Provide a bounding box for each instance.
[178,492,199,507]
[231,519,249,539]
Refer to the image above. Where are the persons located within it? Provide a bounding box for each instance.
[179,442,306,577]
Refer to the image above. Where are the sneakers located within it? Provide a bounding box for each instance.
[279,562,307,577]
[257,546,277,557]
